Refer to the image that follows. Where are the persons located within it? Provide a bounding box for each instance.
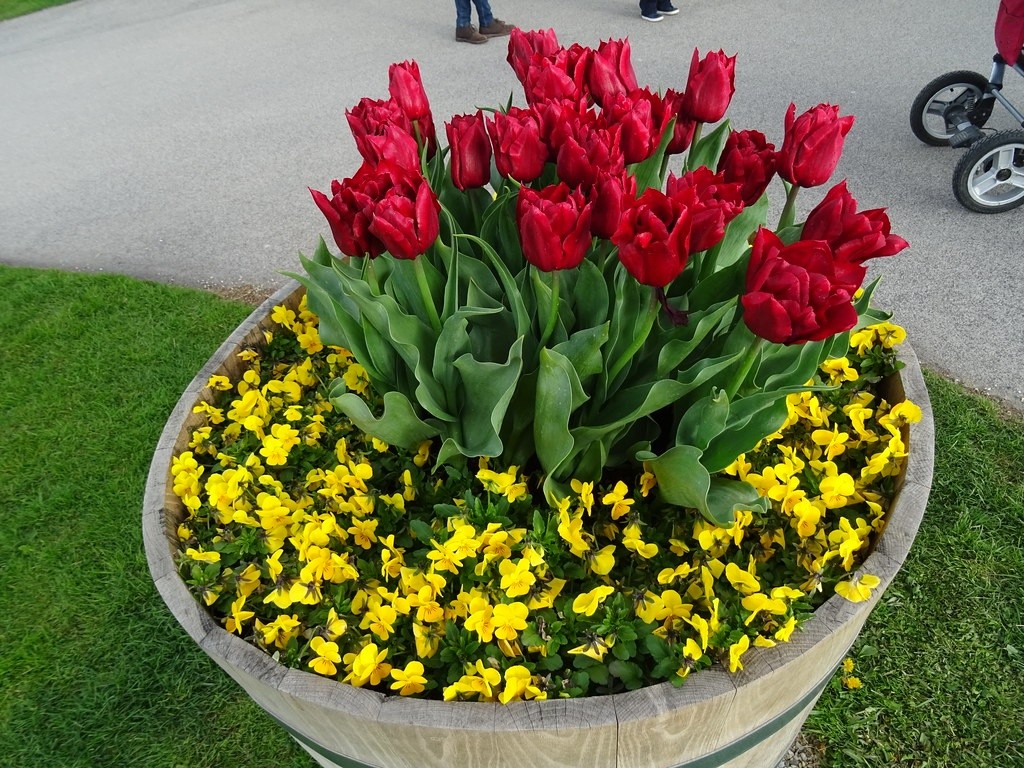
[455,0,516,44]
[639,0,680,22]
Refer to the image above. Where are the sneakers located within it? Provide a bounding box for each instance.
[479,18,516,38]
[642,11,664,22]
[656,5,679,15]
[456,23,488,44]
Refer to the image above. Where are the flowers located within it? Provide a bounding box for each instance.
[165,29,924,705]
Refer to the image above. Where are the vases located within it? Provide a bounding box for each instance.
[143,273,937,768]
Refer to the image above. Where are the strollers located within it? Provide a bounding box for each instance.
[907,0,1024,215]
